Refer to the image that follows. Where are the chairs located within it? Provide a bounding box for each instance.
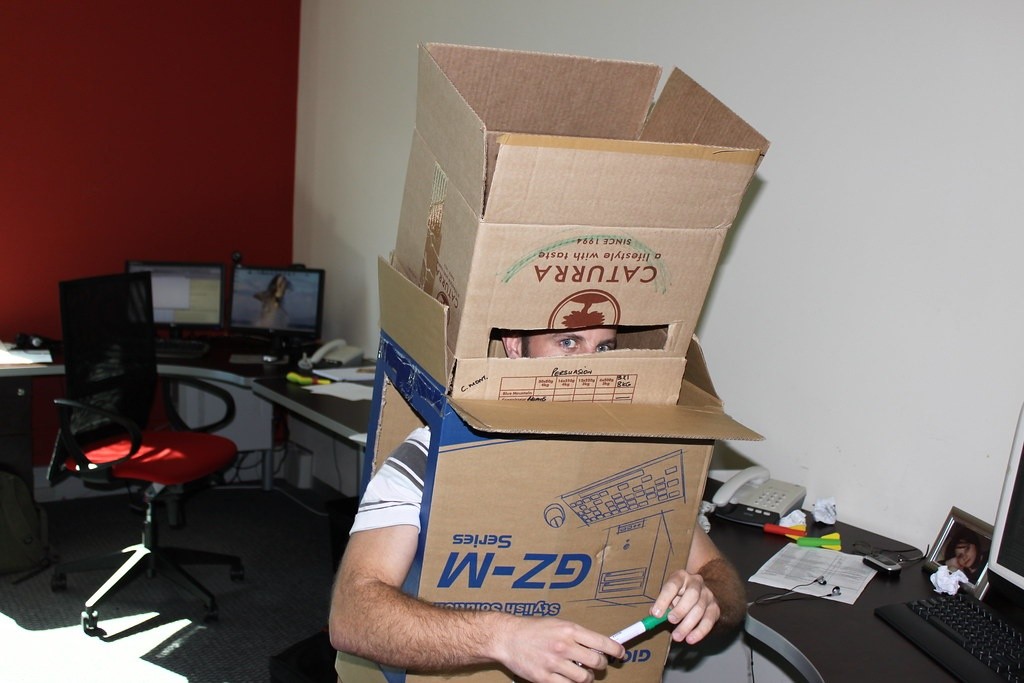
[45,270,247,635]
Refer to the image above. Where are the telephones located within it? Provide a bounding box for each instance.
[708,465,808,528]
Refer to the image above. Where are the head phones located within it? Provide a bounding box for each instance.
[15,334,62,350]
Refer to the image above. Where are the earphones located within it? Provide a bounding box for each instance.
[827,586,841,596]
[815,575,827,585]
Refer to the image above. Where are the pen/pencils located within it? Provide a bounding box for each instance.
[310,337,366,370]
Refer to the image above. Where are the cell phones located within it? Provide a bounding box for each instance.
[862,553,902,575]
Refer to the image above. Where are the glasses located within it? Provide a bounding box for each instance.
[852,541,930,565]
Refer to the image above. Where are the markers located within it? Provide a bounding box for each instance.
[577,608,679,670]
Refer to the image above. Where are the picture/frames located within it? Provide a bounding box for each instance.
[921,505,994,602]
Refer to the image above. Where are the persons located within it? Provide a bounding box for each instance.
[327,328,746,683]
[945,532,990,585]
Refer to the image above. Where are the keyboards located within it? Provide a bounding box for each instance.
[156,338,210,359]
[875,592,1024,683]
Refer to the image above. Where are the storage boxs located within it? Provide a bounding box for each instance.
[334,40,771,683]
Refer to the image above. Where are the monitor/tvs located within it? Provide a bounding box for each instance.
[127,260,229,339]
[988,403,1024,608]
[227,266,325,363]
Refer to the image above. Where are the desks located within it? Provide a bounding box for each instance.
[705,503,1024,683]
[1,337,378,530]
